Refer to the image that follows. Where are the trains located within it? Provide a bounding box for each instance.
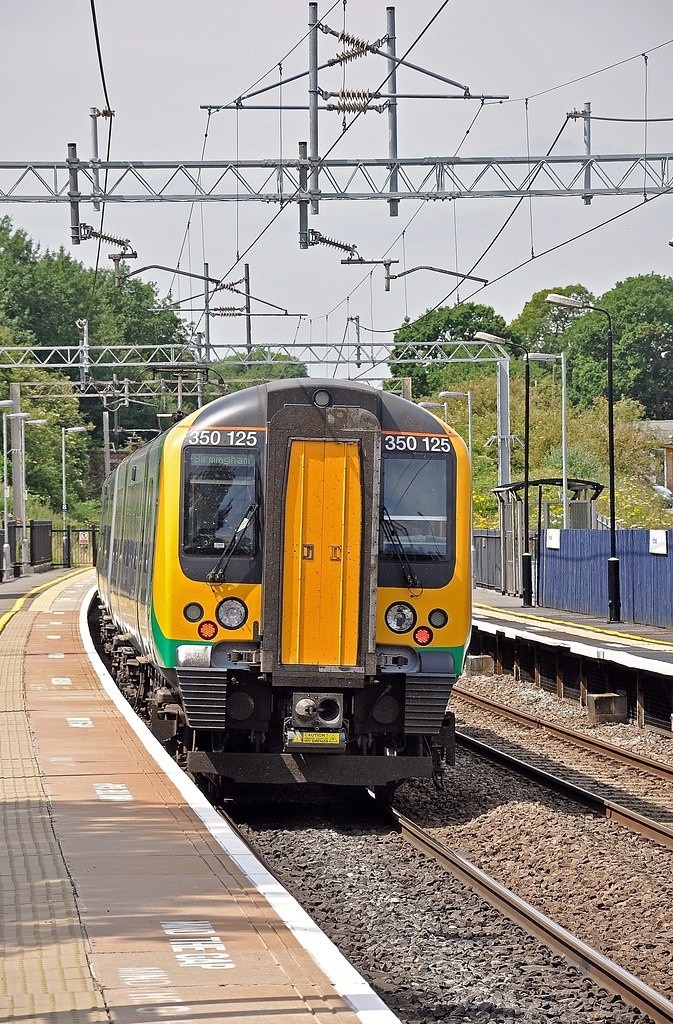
[99,377,474,804]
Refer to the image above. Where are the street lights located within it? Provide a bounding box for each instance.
[436,388,473,479]
[522,350,570,533]
[2,411,31,579]
[544,294,626,626]
[417,401,448,424]
[61,426,88,565]
[472,330,534,609]
[21,418,49,576]
